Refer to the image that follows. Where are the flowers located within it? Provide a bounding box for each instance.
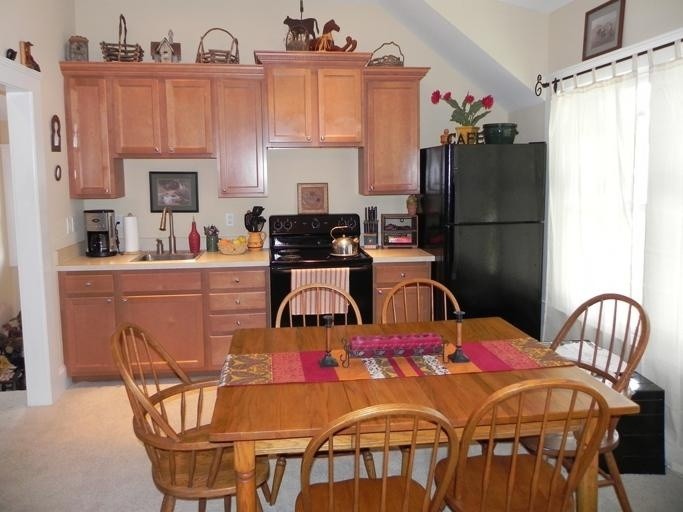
[432,90,494,126]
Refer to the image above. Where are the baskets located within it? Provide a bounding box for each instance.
[365,42,405,66]
[99,15,143,63]
[194,28,239,64]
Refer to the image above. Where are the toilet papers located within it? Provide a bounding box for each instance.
[123,215,138,252]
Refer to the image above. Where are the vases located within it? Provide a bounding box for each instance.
[455,125,479,146]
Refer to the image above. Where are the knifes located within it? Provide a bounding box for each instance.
[364,206,378,234]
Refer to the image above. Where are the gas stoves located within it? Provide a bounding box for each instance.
[268,245,373,264]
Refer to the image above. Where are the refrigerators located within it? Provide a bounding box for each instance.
[418,141,546,341]
[84,210,117,257]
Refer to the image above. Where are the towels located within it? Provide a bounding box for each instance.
[289,268,350,315]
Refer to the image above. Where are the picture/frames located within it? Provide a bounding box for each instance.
[149,171,199,213]
[582,0,625,60]
[297,183,328,214]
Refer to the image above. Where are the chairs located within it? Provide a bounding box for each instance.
[383,278,489,476]
[269,283,376,505]
[295,403,459,512]
[519,294,650,512]
[109,321,270,512]
[435,378,610,512]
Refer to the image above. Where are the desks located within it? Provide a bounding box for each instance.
[209,317,640,512]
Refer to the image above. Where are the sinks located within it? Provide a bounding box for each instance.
[128,250,205,262]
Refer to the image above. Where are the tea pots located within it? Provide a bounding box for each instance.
[329,226,359,255]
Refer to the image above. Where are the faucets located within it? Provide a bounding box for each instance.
[159,205,176,254]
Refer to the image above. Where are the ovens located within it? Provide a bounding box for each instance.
[269,265,373,328]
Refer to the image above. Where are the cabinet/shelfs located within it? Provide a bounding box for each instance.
[358,66,430,194]
[373,262,431,322]
[109,62,216,159]
[60,62,124,198]
[254,50,374,148]
[205,267,270,374]
[58,268,205,380]
[212,64,267,198]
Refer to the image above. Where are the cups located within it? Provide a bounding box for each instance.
[206,235,221,253]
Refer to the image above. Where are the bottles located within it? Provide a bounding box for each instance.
[189,222,200,253]
